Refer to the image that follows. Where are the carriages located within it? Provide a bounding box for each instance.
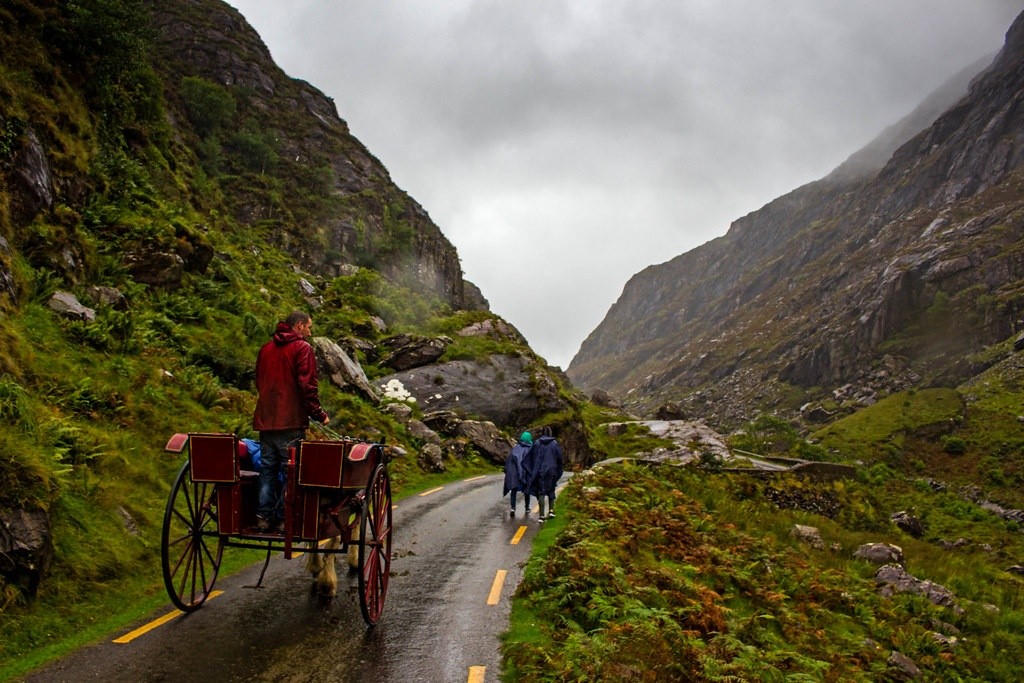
[160,426,407,627]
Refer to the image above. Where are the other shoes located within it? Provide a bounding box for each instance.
[538,517,545,523]
[277,521,285,534]
[526,509,531,513]
[258,520,271,532]
[510,510,515,516]
[548,511,556,517]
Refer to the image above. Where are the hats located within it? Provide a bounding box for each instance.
[521,432,532,442]
[542,426,552,436]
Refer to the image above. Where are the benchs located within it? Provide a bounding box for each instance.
[186,430,258,484]
[299,439,376,490]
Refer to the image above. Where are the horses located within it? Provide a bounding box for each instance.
[305,425,409,597]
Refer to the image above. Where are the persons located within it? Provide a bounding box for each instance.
[521,425,564,524]
[252,310,330,531]
[503,432,531,517]
